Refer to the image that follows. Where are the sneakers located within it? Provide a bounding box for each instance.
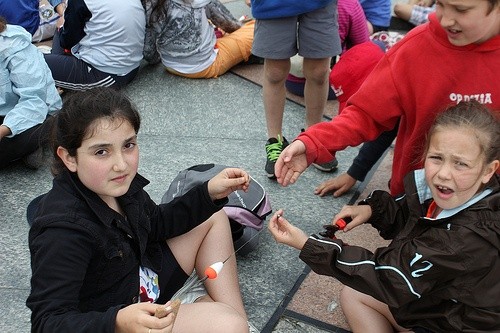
[301,128,338,171]
[264,137,288,178]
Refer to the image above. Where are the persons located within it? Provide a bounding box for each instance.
[274,0,500,198]
[268,98,500,332]
[25,87,250,333]
[0,0,437,172]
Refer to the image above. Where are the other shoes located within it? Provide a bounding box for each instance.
[22,147,43,167]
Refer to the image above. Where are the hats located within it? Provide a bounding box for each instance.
[330,42,385,115]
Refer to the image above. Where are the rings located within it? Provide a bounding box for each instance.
[148,329,151,333]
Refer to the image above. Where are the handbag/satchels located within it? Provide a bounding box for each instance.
[161,164,272,253]
